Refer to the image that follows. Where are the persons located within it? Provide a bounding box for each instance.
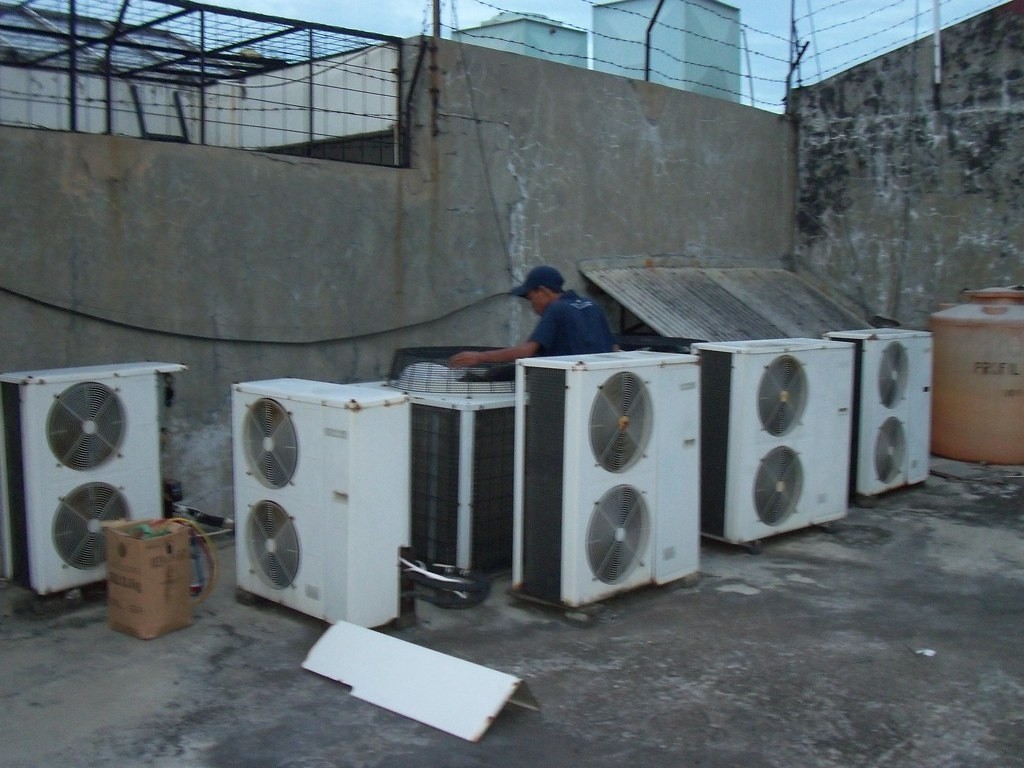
[447,265,620,371]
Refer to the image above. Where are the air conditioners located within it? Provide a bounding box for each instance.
[230,377,410,629]
[505,351,702,611]
[689,338,856,556]
[341,367,516,599]
[0,360,188,597]
[821,327,932,500]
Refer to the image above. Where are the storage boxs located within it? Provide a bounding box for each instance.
[104,518,190,641]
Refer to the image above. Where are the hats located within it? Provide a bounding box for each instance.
[511,266,565,299]
